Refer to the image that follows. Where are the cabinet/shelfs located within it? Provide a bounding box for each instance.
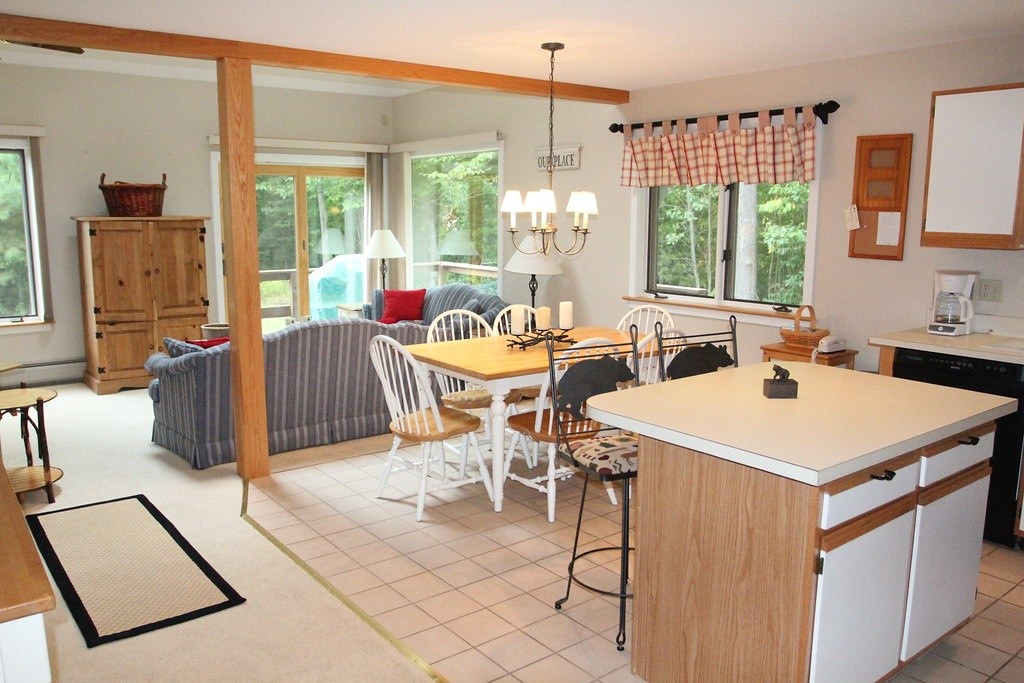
[586,359,1018,683]
[71,215,212,396]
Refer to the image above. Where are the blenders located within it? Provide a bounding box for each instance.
[925,269,980,336]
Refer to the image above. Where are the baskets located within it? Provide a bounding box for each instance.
[780,305,830,348]
[98,172,168,217]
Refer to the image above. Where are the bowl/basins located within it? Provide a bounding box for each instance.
[200,323,230,340]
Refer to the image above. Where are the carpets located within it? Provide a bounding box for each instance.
[22,493,247,648]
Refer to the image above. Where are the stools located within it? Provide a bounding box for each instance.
[545,324,643,651]
[655,315,739,381]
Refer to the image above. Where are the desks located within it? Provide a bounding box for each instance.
[393,328,700,512]
[0,459,56,683]
[336,304,368,320]
[760,343,859,369]
[0,382,63,504]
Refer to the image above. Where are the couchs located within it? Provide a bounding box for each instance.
[363,282,511,340]
[144,318,455,469]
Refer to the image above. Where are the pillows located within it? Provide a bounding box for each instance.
[453,299,480,318]
[374,289,385,321]
[162,337,205,358]
[186,337,230,350]
[381,288,426,323]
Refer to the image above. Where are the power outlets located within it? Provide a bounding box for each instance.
[977,280,1003,303]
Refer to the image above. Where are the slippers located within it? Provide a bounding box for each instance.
[773,305,792,313]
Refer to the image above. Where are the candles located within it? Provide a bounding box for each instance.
[510,306,525,336]
[560,301,574,329]
[535,306,551,332]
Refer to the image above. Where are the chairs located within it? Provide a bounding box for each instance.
[368,306,688,524]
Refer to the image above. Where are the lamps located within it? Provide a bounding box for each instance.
[503,235,564,330]
[364,229,405,289]
[501,43,599,254]
